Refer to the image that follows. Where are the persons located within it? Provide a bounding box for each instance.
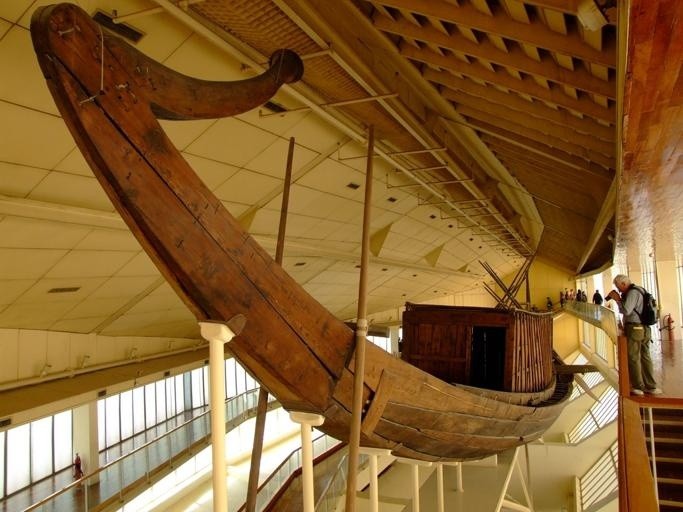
[608,273,663,396]
[73,452,83,477]
[592,289,603,305]
[559,287,587,308]
[546,296,553,311]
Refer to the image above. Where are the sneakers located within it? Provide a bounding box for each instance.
[632,389,644,395]
[645,387,657,394]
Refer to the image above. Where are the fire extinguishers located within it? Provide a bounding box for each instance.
[72,453,83,480]
[662,312,676,341]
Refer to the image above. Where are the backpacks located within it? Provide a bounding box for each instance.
[628,286,658,324]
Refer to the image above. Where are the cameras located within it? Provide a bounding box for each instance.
[605,290,619,301]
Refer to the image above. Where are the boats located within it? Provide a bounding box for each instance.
[30,4,574,461]
